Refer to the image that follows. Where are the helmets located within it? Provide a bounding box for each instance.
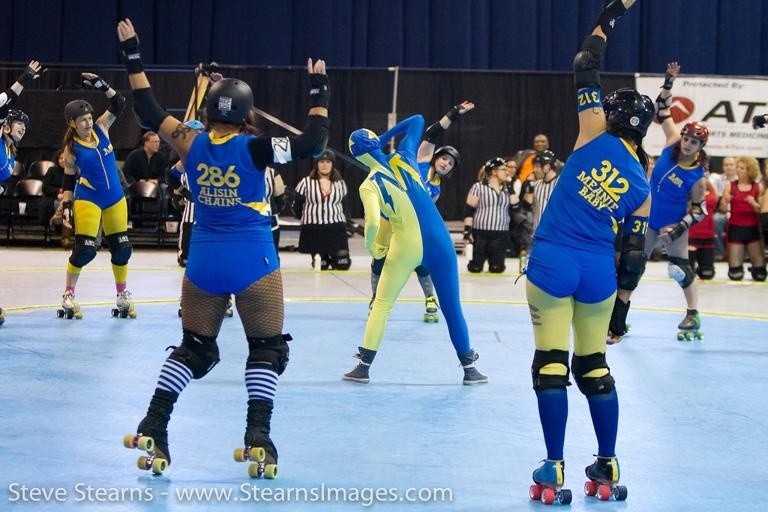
[434,146,462,169]
[186,119,206,130]
[600,87,655,135]
[486,158,506,172]
[316,149,335,163]
[64,100,94,124]
[533,149,558,164]
[206,77,254,125]
[7,108,31,129]
[680,121,710,143]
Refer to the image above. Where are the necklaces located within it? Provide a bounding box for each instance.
[709,157,740,260]
[725,158,767,281]
[685,160,725,279]
[271,169,286,261]
[759,158,768,263]
[601,62,709,330]
[126,130,165,229]
[343,115,490,386]
[513,135,563,179]
[0,60,43,328]
[505,155,521,198]
[524,3,653,490]
[463,157,519,277]
[42,148,75,249]
[115,16,331,479]
[169,121,232,320]
[531,150,562,236]
[293,150,354,271]
[49,71,136,320]
[516,158,539,275]
[369,100,475,322]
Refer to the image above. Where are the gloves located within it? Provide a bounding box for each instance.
[18,66,36,87]
[120,34,144,73]
[89,76,110,92]
[306,74,331,108]
[446,104,465,122]
[597,1,628,37]
[62,200,73,229]
[663,73,676,90]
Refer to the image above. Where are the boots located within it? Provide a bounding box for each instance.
[49,208,62,231]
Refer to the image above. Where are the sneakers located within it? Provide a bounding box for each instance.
[463,367,491,384]
[344,361,370,383]
[60,236,74,251]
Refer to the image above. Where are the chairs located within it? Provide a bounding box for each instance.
[27,160,56,180]
[10,177,54,248]
[159,185,188,248]
[12,161,27,176]
[126,182,159,246]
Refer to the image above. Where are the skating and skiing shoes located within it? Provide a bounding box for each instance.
[677,315,703,340]
[234,428,278,479]
[424,297,440,323]
[530,460,572,505]
[124,418,171,473]
[57,291,83,319]
[111,292,137,318]
[607,322,631,344]
[585,457,628,500]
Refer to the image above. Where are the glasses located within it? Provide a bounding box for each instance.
[507,165,518,169]
